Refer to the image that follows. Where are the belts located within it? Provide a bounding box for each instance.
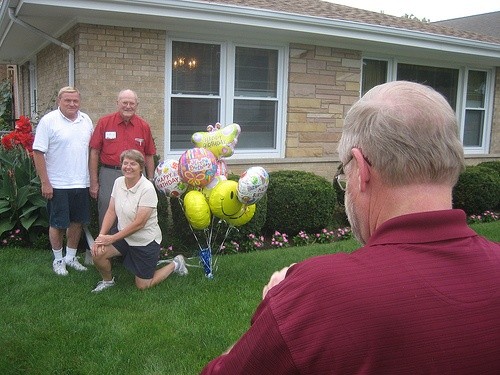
[101,163,121,170]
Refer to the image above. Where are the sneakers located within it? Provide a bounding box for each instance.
[64,257,87,271]
[90,276,116,293]
[173,255,188,278]
[52,259,69,277]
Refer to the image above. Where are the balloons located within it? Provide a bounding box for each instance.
[154,123,270,230]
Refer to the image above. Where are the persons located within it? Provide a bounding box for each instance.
[89,90,156,235]
[32,86,94,275]
[91,149,188,293]
[200,81,500,375]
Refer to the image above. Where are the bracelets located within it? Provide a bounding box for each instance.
[148,178,154,181]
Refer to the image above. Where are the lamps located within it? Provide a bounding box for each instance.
[173,58,197,71]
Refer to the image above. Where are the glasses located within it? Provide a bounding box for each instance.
[119,102,136,107]
[335,153,372,191]
